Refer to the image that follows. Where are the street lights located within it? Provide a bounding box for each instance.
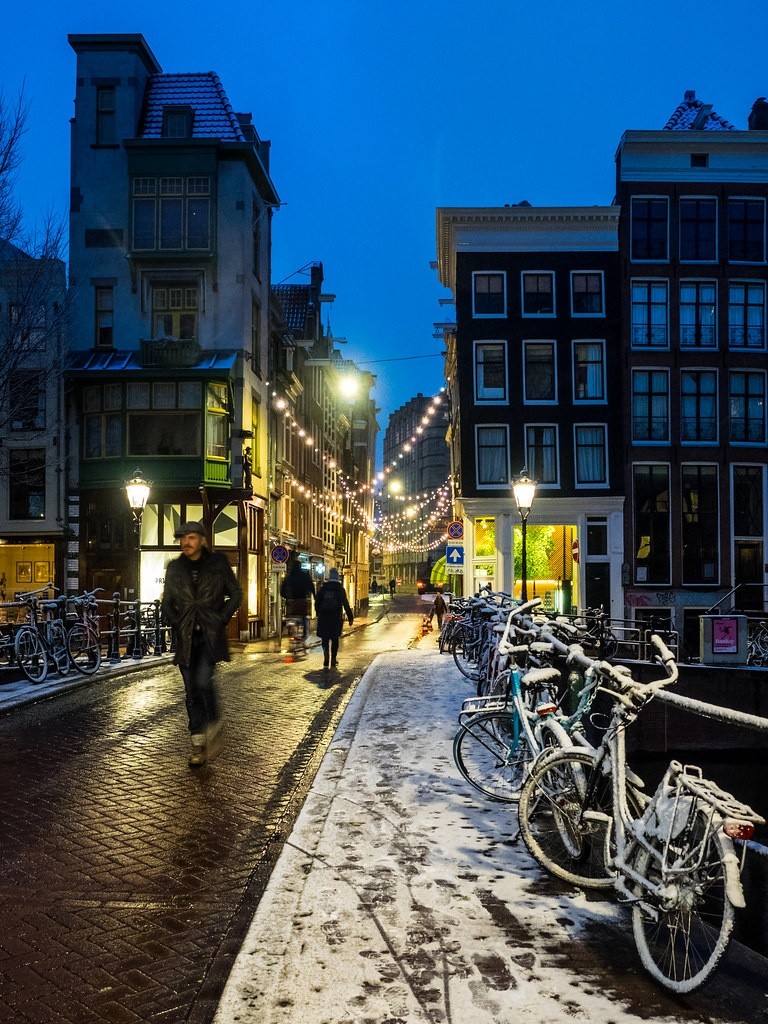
[118,466,156,660]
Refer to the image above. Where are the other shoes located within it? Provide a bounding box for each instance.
[323,663,328,666]
[330,661,338,666]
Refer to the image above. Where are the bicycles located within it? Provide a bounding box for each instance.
[435,582,768,1001]
[14,581,107,686]
[510,464,540,616]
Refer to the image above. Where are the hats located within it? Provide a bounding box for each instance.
[173,521,206,538]
[328,568,338,578]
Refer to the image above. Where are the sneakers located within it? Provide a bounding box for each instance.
[188,745,207,765]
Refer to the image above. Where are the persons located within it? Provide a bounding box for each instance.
[244,447,253,488]
[389,579,396,594]
[316,567,354,670]
[162,521,245,763]
[434,592,448,628]
[372,579,377,593]
[281,561,315,638]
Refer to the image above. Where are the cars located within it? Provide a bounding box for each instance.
[417,576,438,595]
